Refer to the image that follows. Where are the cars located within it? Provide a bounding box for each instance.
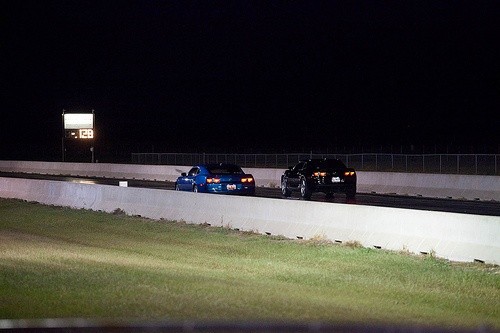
[175,162,256,196]
[281,158,357,199]
